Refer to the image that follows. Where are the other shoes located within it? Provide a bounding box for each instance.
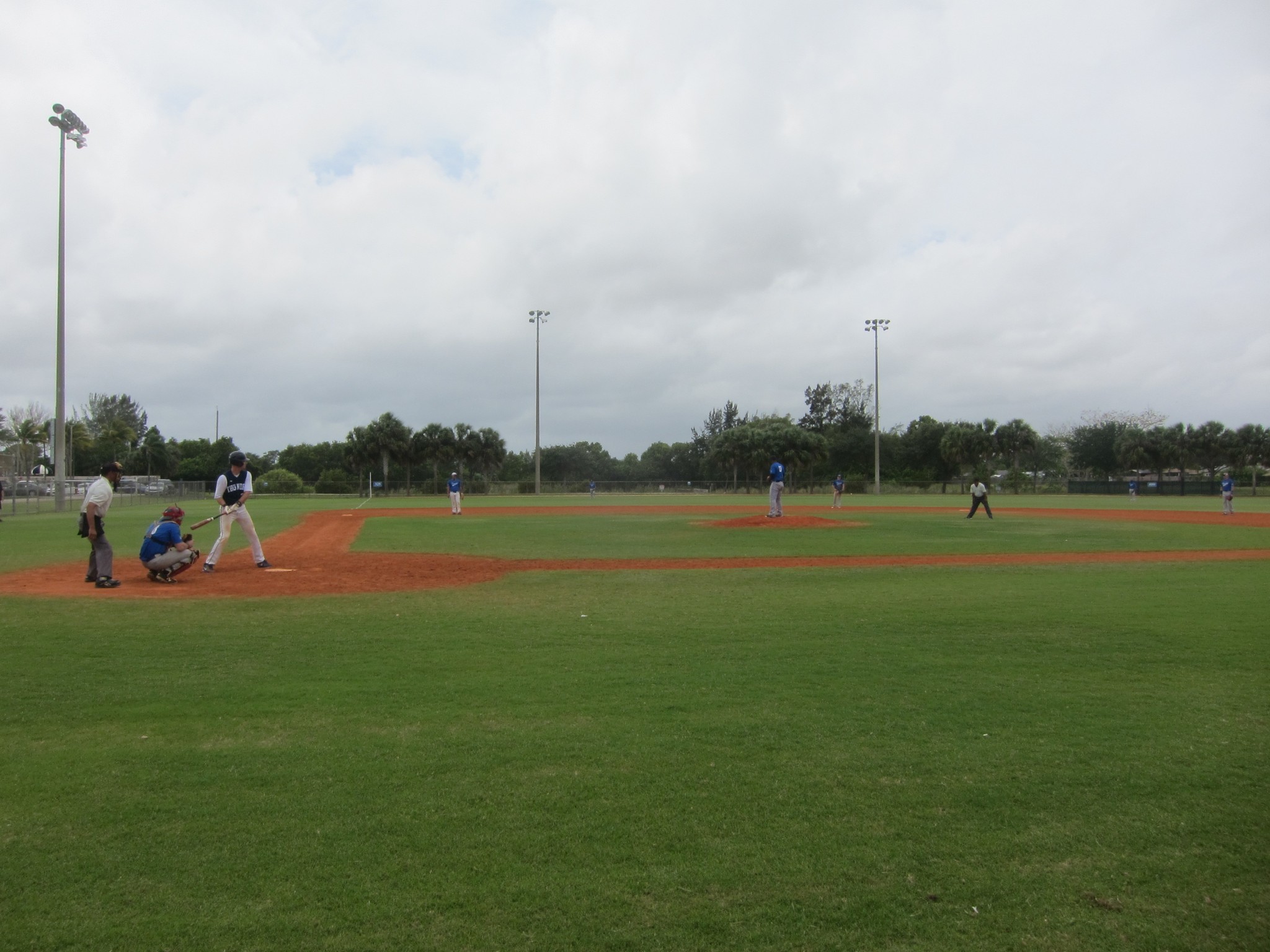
[764,514,776,518]
[146,569,160,583]
[831,506,835,508]
[1231,512,1233,515]
[776,513,784,517]
[1222,512,1227,515]
[457,512,462,515]
[452,512,457,515]
[838,507,841,509]
[155,569,179,583]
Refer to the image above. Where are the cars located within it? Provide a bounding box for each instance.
[0,480,50,496]
[77,483,92,494]
[145,479,175,496]
[116,483,147,494]
[46,483,77,496]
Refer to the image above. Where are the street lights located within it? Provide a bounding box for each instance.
[865,319,890,494]
[529,310,550,493]
[48,103,89,513]
[67,423,75,477]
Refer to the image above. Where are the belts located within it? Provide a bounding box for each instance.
[776,480,780,482]
[144,551,164,563]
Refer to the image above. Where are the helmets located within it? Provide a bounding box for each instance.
[156,506,184,522]
[229,451,250,466]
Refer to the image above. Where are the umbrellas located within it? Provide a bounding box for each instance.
[32,464,53,475]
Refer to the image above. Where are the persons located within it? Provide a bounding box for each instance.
[831,474,844,508]
[202,451,273,573]
[78,461,124,588]
[447,472,464,515]
[1129,479,1136,496]
[966,477,993,519]
[589,480,596,499]
[139,506,200,583]
[1220,473,1234,515]
[767,455,785,517]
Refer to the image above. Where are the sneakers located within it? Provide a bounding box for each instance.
[202,562,216,573]
[94,576,122,588]
[85,575,97,583]
[257,559,273,568]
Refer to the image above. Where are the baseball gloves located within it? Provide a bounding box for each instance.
[460,493,464,500]
[182,533,192,543]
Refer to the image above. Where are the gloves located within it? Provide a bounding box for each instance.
[223,503,231,515]
[229,500,244,513]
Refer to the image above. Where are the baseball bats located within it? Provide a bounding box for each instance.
[190,512,227,530]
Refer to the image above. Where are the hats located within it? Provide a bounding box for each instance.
[102,461,125,473]
[1223,473,1229,476]
[452,472,457,476]
[837,474,842,477]
